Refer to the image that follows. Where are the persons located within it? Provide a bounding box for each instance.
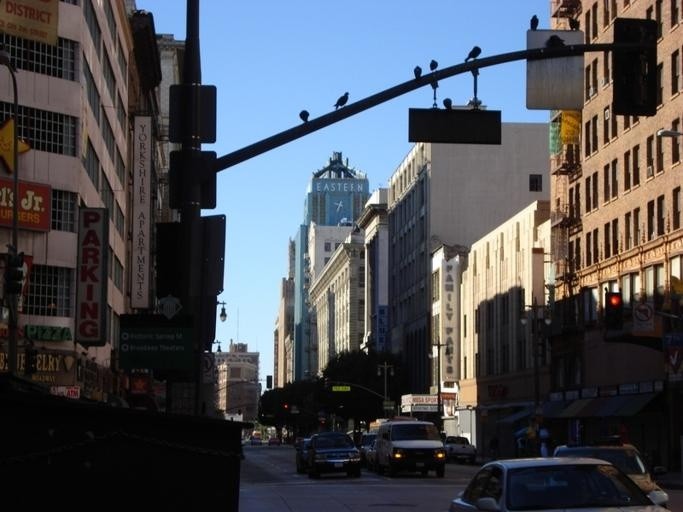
[489,431,501,456]
[518,437,525,457]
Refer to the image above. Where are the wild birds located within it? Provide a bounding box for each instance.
[413,64,422,79]
[529,13,540,29]
[429,58,438,72]
[443,97,453,110]
[465,46,482,61]
[299,109,309,122]
[333,91,351,108]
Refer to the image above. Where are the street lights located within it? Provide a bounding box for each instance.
[376,361,395,419]
[426,334,451,432]
[212,339,222,355]
[0,46,25,378]
[654,128,683,139]
[521,295,553,435]
[216,300,228,323]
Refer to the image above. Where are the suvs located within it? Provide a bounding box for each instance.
[552,433,669,509]
[305,432,362,480]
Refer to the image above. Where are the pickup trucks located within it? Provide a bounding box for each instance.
[442,433,477,464]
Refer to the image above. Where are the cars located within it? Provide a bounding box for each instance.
[251,430,262,445]
[268,437,281,446]
[291,430,379,475]
[445,455,674,512]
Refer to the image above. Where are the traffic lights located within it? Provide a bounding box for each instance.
[1,250,25,296]
[24,346,38,376]
[603,292,624,334]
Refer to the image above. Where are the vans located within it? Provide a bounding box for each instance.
[375,416,449,480]
